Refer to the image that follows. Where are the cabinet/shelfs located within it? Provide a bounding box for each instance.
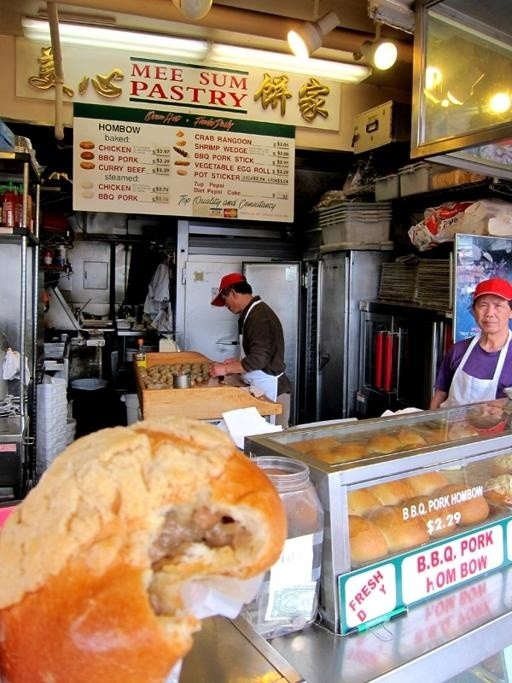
[356,300,446,417]
[0,149,46,499]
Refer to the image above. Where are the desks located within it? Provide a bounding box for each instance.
[266,564,512,681]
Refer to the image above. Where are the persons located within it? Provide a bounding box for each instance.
[428,276,512,413]
[207,273,292,429]
[465,402,508,437]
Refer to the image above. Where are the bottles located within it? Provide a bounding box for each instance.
[138,338,146,352]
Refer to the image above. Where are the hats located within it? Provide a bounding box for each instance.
[211,273,245,306]
[474,278,512,301]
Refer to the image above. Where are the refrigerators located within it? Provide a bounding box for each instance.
[243,250,381,421]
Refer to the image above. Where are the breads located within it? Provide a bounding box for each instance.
[139,361,215,389]
[0,416,287,682]
[287,421,511,562]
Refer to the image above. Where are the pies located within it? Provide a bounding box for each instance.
[174,160,191,175]
[175,130,187,147]
[79,140,95,200]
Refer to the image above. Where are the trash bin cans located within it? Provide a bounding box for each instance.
[70,378,108,438]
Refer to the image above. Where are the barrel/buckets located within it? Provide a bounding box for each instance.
[240,454,326,640]
[122,393,144,427]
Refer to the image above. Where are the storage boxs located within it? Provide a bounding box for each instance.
[352,101,411,155]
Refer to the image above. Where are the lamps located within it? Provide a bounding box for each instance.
[360,19,397,71]
[286,0,340,61]
